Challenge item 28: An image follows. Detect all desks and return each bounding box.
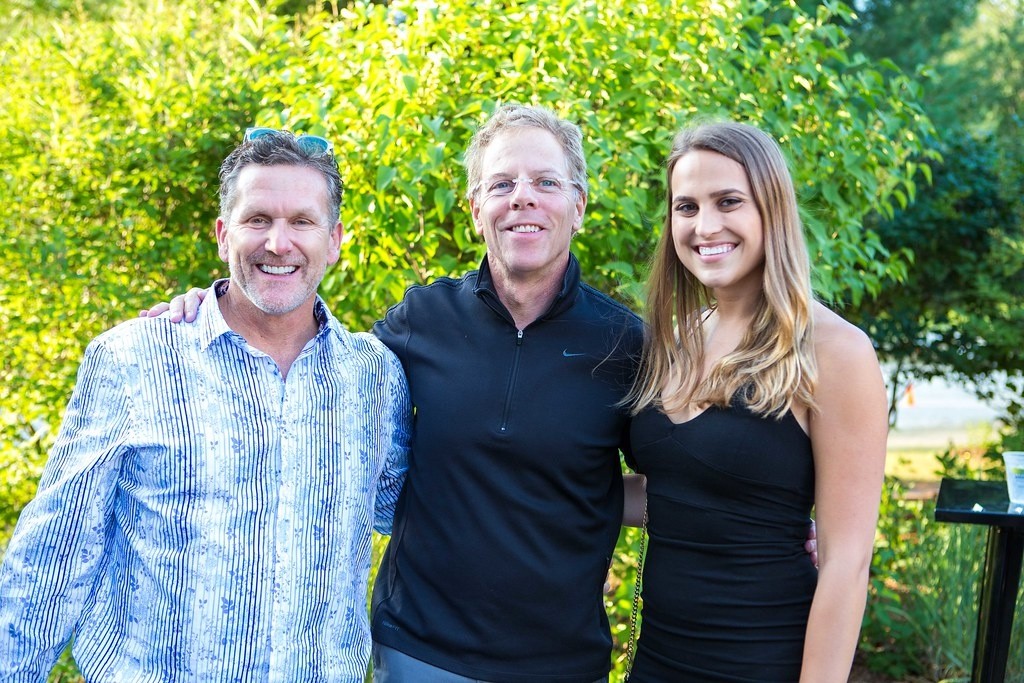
[934,476,1024,683]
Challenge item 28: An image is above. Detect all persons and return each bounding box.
[620,122,888,683]
[140,103,818,683]
[0,128,414,683]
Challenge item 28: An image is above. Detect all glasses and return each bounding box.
[474,173,581,195]
[243,127,334,163]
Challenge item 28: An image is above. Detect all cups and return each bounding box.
[1002,452,1024,503]
